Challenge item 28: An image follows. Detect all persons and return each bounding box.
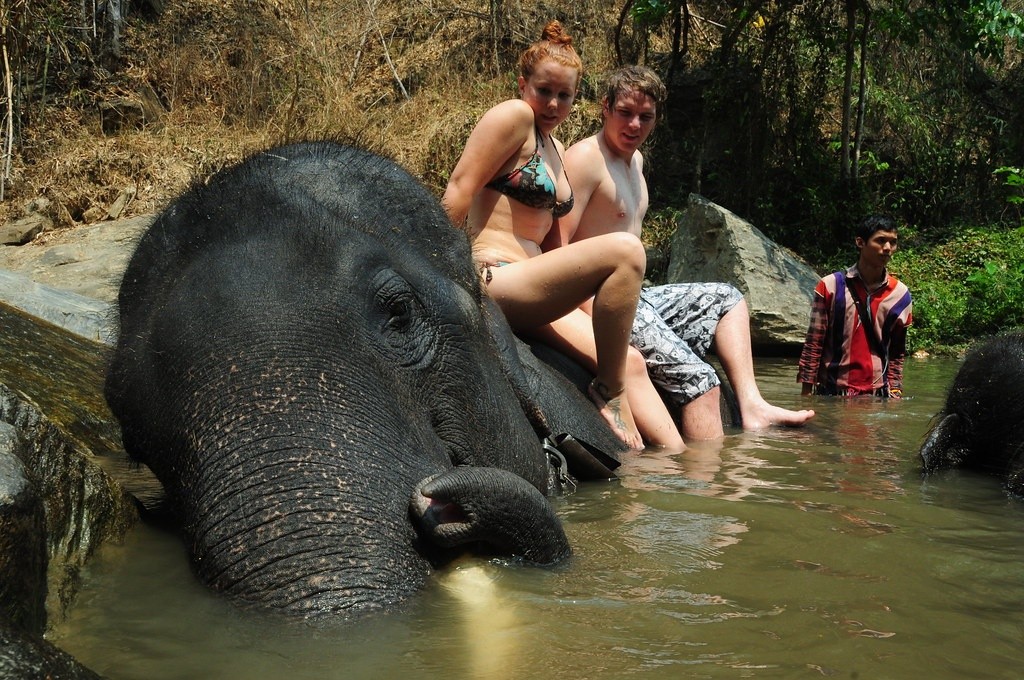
[438,21,689,455]
[538,63,814,442]
[795,210,915,402]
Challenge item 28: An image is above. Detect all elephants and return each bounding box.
[103,126,1023,640]
